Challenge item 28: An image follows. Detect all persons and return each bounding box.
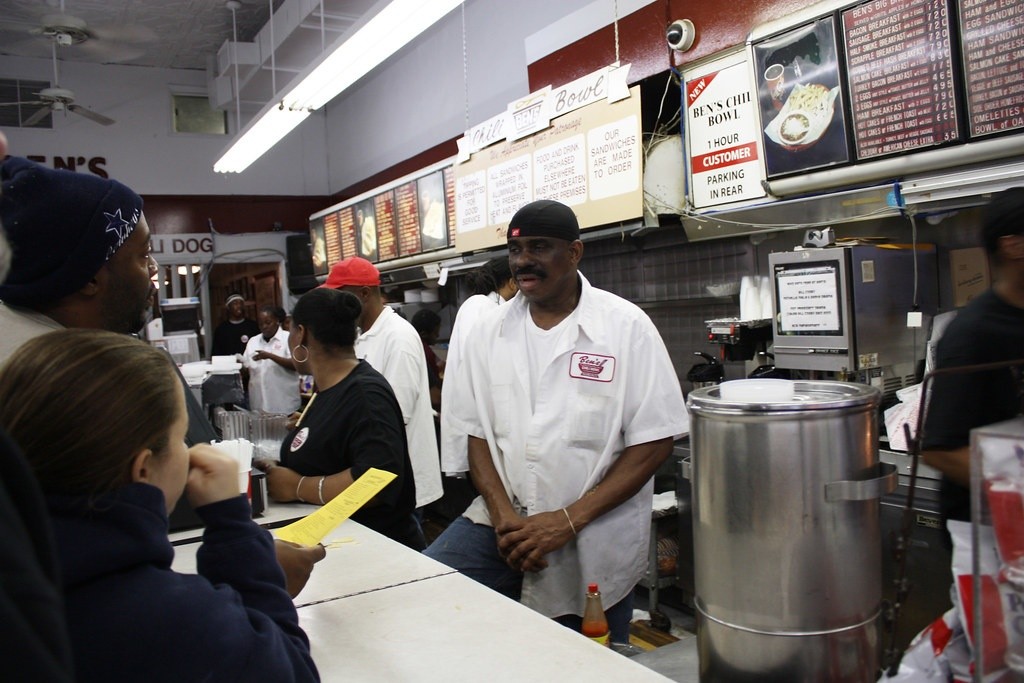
[205,255,519,554]
[0,157,325,601]
[918,185,1024,609]
[419,200,691,645]
[1,328,321,683]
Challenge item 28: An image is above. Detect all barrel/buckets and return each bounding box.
[684,378,901,634]
[693,596,888,683]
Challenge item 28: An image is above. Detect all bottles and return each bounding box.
[581,585,611,649]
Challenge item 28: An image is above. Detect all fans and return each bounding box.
[0,37,117,126]
[3,10,154,69]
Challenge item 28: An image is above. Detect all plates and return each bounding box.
[404,286,439,305]
[779,112,812,144]
[361,217,376,256]
[422,202,445,240]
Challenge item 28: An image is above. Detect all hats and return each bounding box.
[309,257,381,291]
[225,291,245,310]
[0,132,144,306]
[507,200,580,241]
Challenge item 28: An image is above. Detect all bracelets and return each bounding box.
[319,475,325,505]
[296,475,306,503]
[562,507,577,536]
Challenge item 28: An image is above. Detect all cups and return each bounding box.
[237,470,253,512]
[764,63,787,112]
[421,191,430,212]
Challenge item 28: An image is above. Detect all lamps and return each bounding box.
[209,0,466,174]
